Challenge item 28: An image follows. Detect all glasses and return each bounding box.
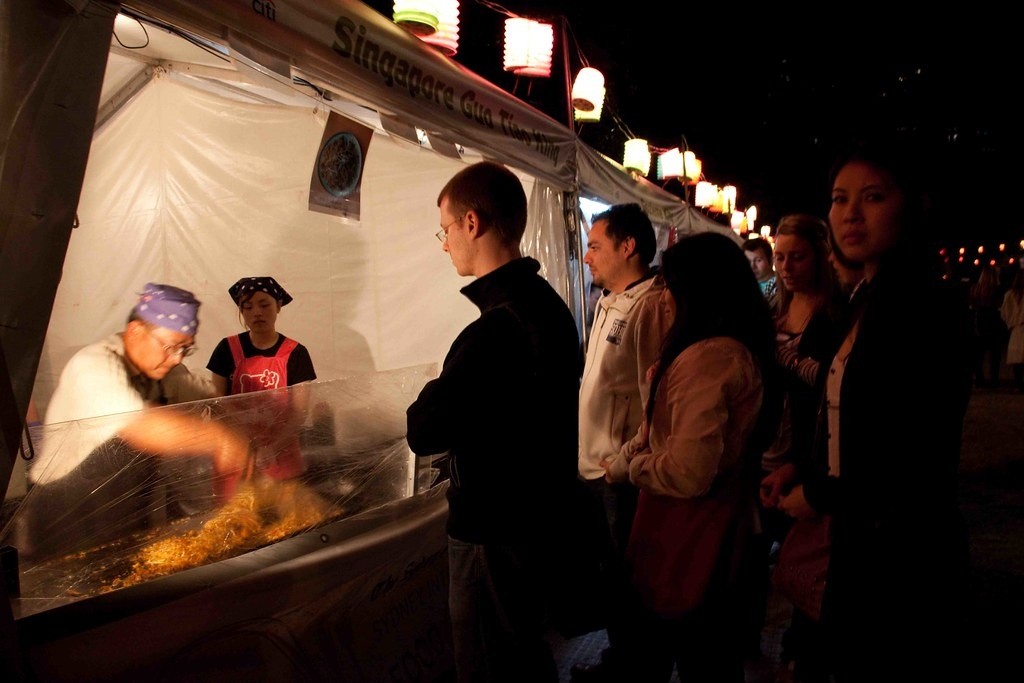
[143,326,197,357]
[435,214,469,244]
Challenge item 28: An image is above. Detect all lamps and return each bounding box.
[623,138,771,240]
[571,67,606,124]
[502,17,553,78]
[392,1,460,57]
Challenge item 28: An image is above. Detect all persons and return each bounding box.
[28,281,252,566]
[802,146,977,683]
[408,163,1024,683]
[207,278,316,510]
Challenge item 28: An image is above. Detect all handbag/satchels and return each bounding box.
[620,481,741,616]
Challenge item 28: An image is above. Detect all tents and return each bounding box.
[570,138,752,359]
[0,0,577,683]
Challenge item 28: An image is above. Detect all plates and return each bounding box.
[319,132,361,197]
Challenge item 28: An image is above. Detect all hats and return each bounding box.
[137,282,200,334]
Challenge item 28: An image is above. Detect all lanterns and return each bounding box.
[624,138,652,177]
[393,0,460,55]
[504,18,554,77]
[572,67,606,122]
[657,147,757,235]
